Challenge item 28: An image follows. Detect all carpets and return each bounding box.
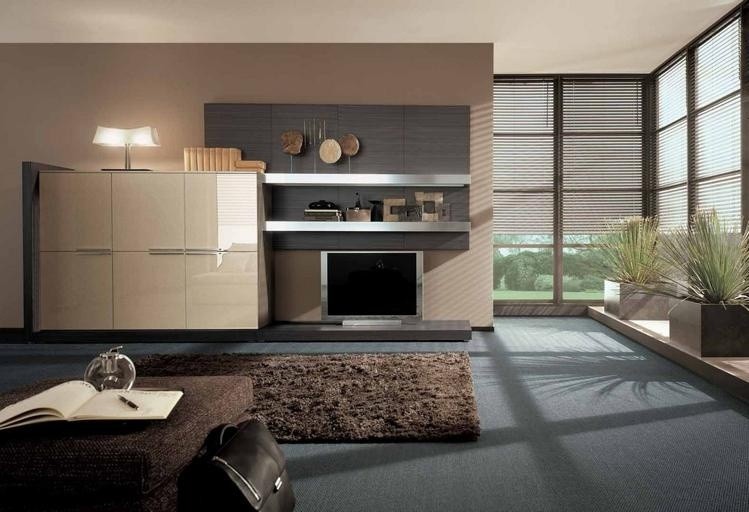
[121,351,481,444]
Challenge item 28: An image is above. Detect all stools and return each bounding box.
[0,374,254,512]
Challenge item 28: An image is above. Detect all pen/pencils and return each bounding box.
[117,394,139,410]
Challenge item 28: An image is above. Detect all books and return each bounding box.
[235,166,265,173]
[0,379,184,431]
[183,147,242,172]
[234,159,266,169]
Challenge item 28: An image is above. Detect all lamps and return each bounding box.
[92,125,162,171]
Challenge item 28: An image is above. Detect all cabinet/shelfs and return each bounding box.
[262,173,473,233]
[37,170,272,332]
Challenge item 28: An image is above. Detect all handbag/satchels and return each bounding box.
[208,417,296,512]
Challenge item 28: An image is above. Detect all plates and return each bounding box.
[318,134,359,164]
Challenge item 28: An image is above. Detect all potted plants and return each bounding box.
[585,207,748,358]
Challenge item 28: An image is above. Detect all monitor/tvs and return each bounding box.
[320,250,423,326]
